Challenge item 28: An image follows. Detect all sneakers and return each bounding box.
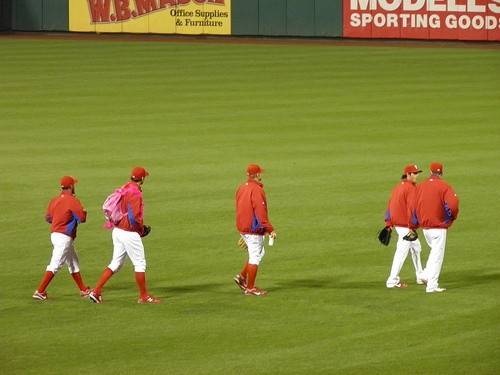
[234,274,247,291]
[394,283,406,288]
[89,291,103,304]
[426,286,446,292]
[245,286,267,295]
[138,296,160,303]
[420,276,427,284]
[79,289,93,299]
[32,290,46,300]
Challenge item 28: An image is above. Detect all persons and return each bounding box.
[384,165,424,289]
[88,167,160,303]
[32,176,94,300]
[409,162,459,292]
[233,164,277,295]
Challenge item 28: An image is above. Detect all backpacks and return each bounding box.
[103,187,140,227]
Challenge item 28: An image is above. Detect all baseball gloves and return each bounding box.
[142,225,152,237]
[237,237,248,250]
[402,230,419,242]
[377,226,392,246]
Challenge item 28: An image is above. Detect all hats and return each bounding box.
[60,176,78,188]
[405,166,422,175]
[429,162,443,173]
[131,167,149,180]
[247,165,264,175]
